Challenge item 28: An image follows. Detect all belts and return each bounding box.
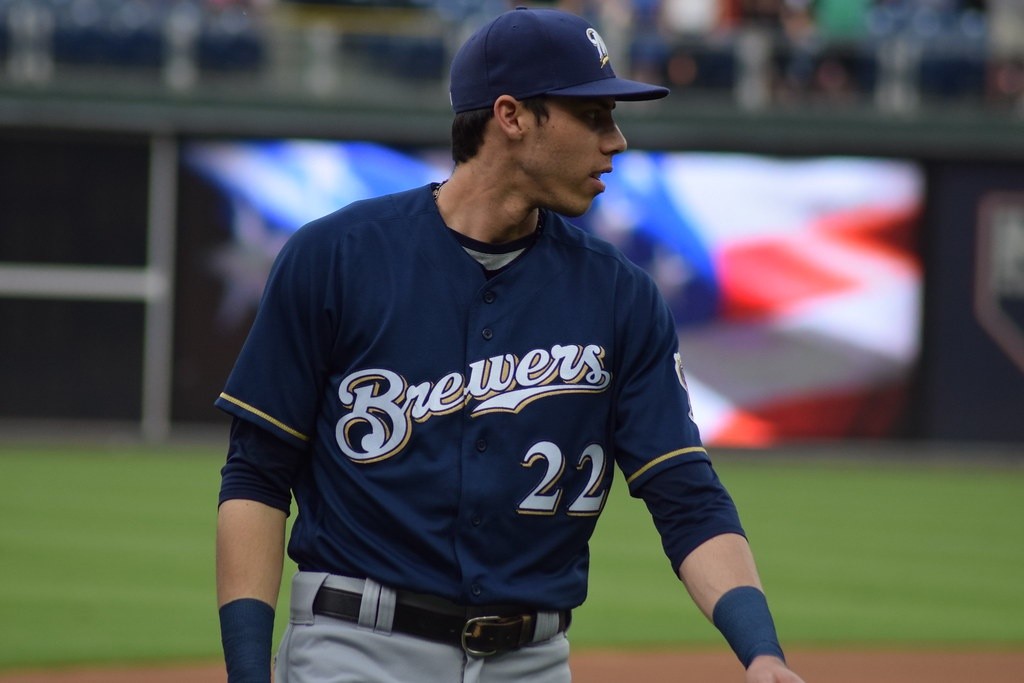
[317,587,573,657]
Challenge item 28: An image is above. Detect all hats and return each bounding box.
[448,7,671,108]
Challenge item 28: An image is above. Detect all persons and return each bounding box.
[214,9,804,683]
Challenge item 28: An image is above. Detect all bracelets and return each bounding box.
[710,585,786,670]
[219,598,275,683]
